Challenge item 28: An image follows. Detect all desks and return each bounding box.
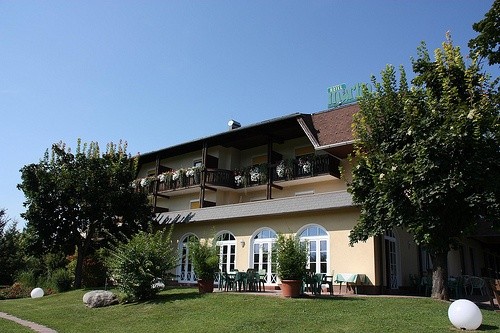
[227,273,258,291]
[334,273,369,296]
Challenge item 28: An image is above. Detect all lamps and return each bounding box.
[240,240,245,248]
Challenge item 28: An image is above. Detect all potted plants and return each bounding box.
[55,268,73,292]
[183,226,223,294]
[273,227,311,297]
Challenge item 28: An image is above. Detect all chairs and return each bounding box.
[409,271,487,299]
[305,271,333,295]
[218,269,267,292]
